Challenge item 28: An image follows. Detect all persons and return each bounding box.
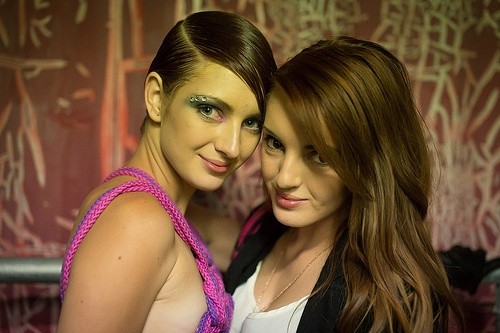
[56,10,276,333]
[220,35,464,333]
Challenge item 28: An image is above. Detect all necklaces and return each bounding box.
[252,241,338,312]
